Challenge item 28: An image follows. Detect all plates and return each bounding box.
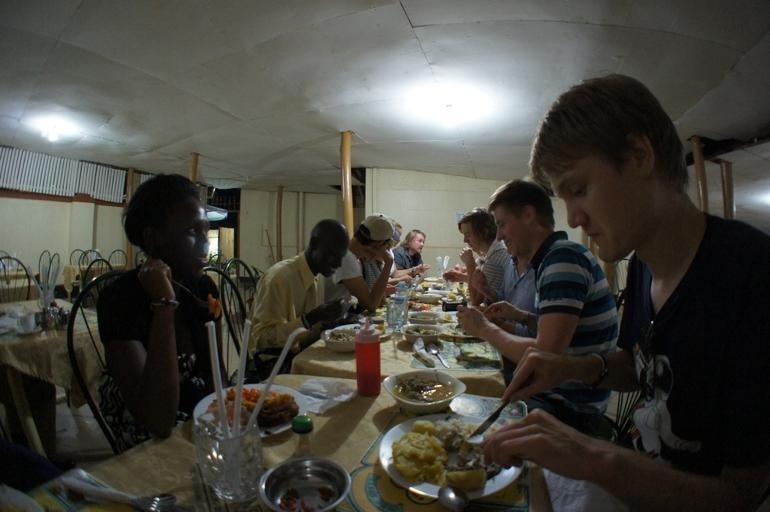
[17,327,43,335]
[379,416,529,503]
[192,385,308,440]
[323,278,490,368]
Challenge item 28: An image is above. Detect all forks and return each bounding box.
[60,475,176,512]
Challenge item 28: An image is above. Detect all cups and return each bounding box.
[19,313,36,332]
[192,405,266,504]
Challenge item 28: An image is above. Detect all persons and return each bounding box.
[96,173,229,447]
[319,214,396,322]
[454,249,485,273]
[362,218,431,296]
[472,244,537,339]
[455,177,618,434]
[250,218,354,382]
[477,73,770,512]
[442,207,511,307]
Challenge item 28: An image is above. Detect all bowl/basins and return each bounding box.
[258,455,352,512]
[383,369,468,415]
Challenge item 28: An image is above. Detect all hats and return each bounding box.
[361,213,394,241]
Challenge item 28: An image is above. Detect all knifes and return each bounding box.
[468,390,519,437]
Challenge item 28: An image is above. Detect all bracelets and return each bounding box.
[583,348,611,393]
[518,310,533,327]
[298,310,311,329]
[148,295,182,314]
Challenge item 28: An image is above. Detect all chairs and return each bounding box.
[201,270,248,385]
[0,246,147,303]
[219,258,257,360]
[588,288,644,450]
[66,269,136,456]
